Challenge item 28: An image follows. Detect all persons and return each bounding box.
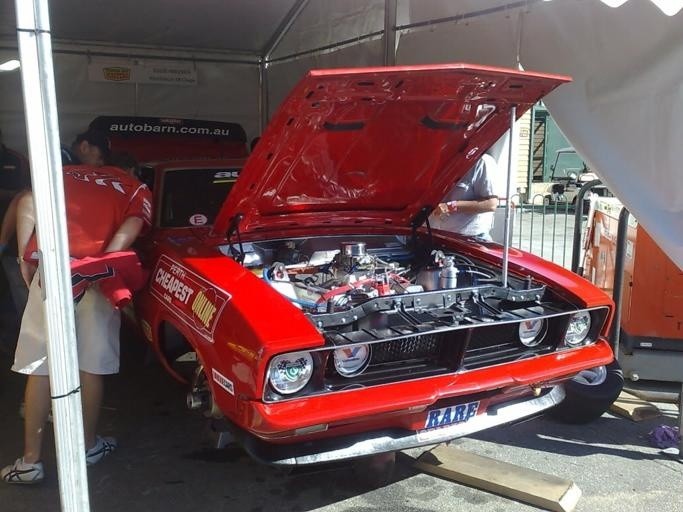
[0,154,154,484]
[0,130,112,423]
[431,153,502,244]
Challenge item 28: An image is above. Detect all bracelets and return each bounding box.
[447,199,459,217]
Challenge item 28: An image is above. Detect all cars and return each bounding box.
[71,62,624,478]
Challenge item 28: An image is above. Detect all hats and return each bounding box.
[71,129,111,148]
[61,148,79,164]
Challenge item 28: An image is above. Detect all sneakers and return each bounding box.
[85,436,117,466]
[20,401,52,423]
[1,457,45,485]
